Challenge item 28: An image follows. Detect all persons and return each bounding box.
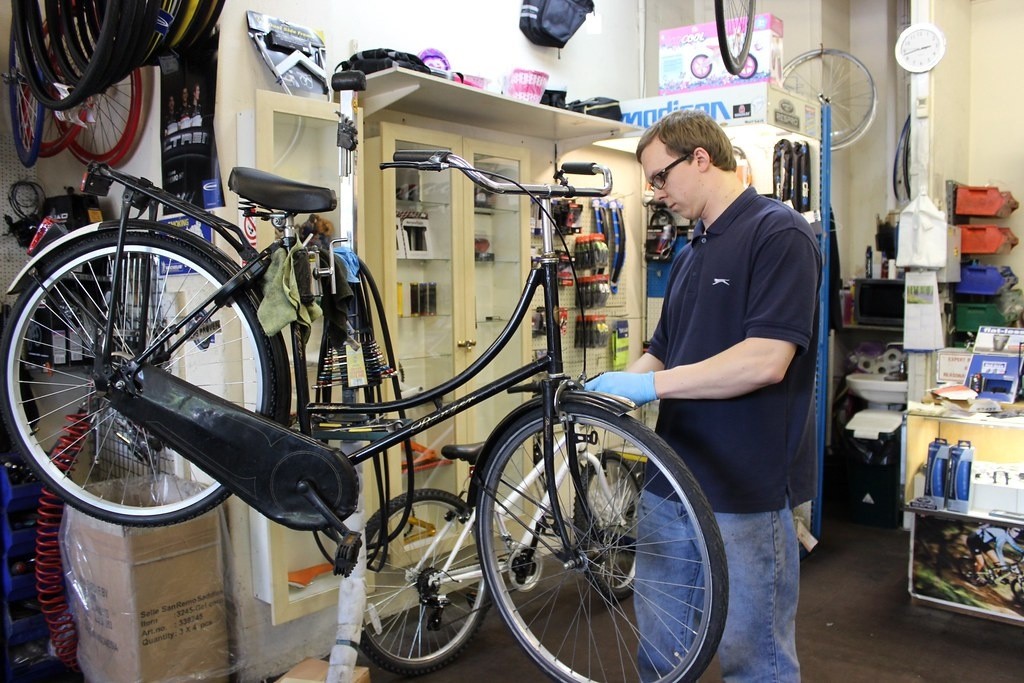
[967,525,1024,581]
[581,108,824,683]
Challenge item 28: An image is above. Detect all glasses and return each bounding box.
[649,145,713,190]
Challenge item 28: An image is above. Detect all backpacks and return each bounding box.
[519,0,595,60]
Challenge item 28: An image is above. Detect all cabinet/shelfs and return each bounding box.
[254,67,643,626]
[901,347,1024,525]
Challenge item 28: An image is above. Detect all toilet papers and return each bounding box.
[856,348,901,377]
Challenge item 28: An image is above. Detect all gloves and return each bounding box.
[582,370,659,410]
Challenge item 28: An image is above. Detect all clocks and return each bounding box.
[894,24,946,73]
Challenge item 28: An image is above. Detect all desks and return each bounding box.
[825,324,903,454]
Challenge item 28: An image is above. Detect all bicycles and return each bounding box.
[0,149,728,683]
[688,27,758,80]
[956,553,1024,607]
[352,379,642,678]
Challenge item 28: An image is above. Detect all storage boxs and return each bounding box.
[955,302,1011,332]
[658,14,784,95]
[273,658,371,683]
[955,187,1018,218]
[65,473,230,683]
[955,259,1017,295]
[960,224,1021,255]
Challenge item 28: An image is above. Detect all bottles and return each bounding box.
[925,438,951,509]
[946,440,973,512]
